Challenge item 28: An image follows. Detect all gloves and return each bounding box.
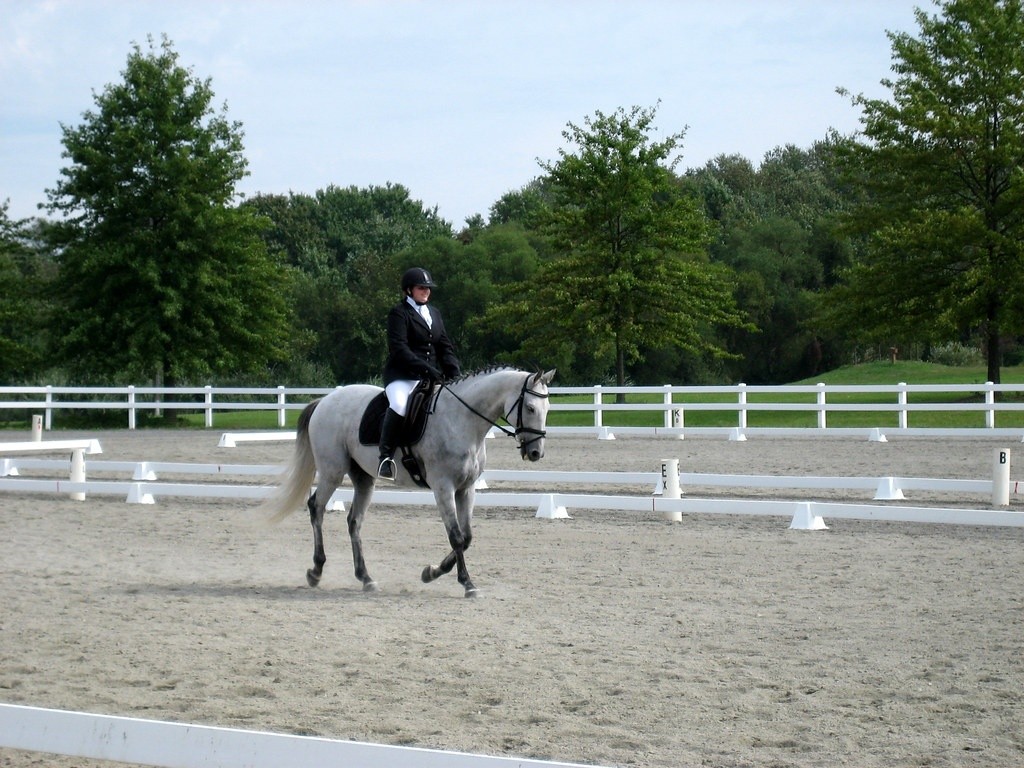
[429,367,443,380]
[443,355,460,375]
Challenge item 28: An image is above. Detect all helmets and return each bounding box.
[401,267,437,306]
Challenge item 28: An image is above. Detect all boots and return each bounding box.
[377,407,406,478]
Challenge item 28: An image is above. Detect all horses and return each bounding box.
[248,366,557,598]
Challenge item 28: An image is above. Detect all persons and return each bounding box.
[377,267,461,478]
